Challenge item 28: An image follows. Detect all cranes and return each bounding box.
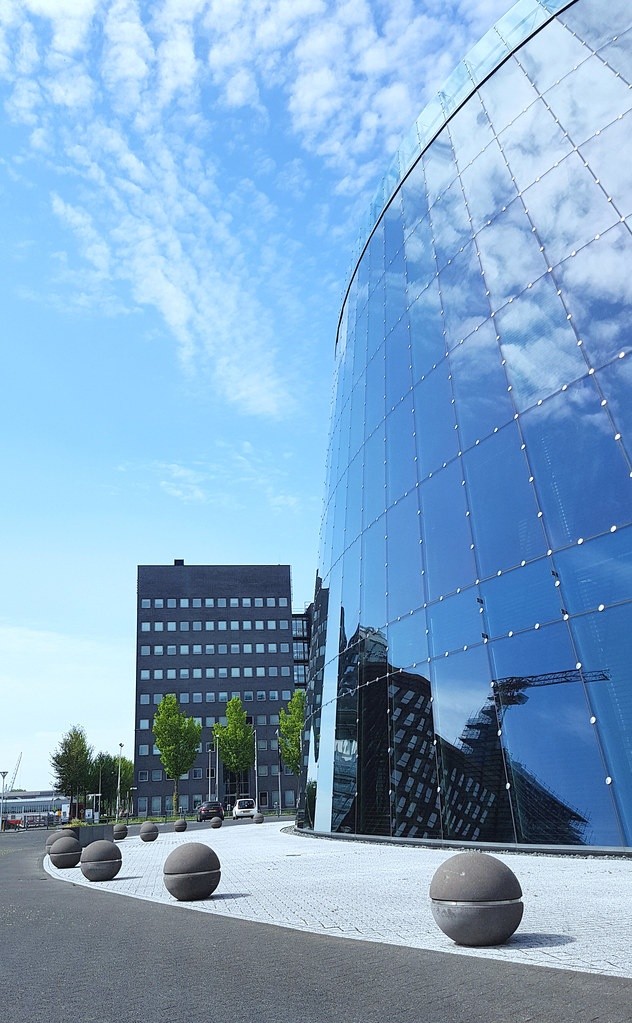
[487,665,611,736]
[7,752,24,792]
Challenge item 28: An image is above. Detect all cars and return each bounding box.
[196,800,225,822]
[231,799,255,820]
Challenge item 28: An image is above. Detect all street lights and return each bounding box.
[118,742,124,806]
[215,734,219,801]
[208,749,212,802]
[0,771,9,820]
